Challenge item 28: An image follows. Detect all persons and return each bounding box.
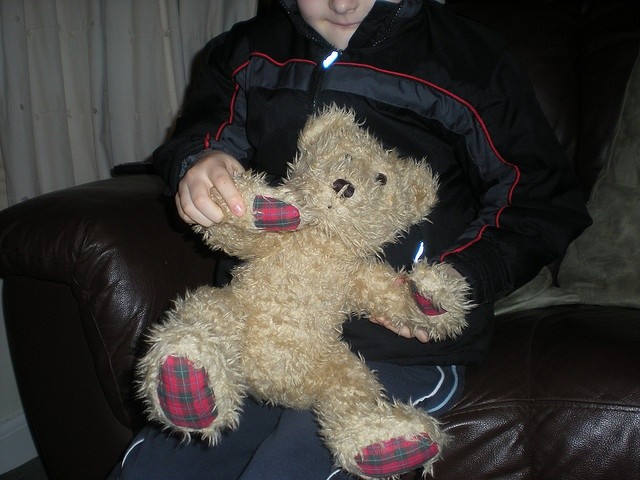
[111,0,593,480]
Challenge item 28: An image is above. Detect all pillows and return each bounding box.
[555,49,640,304]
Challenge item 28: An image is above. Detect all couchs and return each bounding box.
[1,2,640,480]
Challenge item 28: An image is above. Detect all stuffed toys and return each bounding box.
[137,104,478,480]
